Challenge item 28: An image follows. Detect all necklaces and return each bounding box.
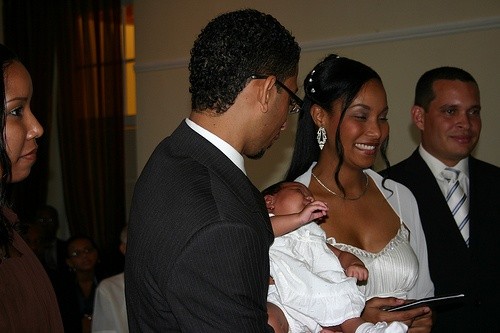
[311,171,369,201]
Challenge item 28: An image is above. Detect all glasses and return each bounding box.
[68,246,93,258]
[252,74,304,114]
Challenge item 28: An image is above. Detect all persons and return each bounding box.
[122,8,309,333]
[0,42,65,333]
[19,205,103,333]
[88,226,129,333]
[377,66,500,333]
[285,52,436,333]
[260,179,409,333]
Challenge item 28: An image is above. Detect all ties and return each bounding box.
[440,168,471,249]
